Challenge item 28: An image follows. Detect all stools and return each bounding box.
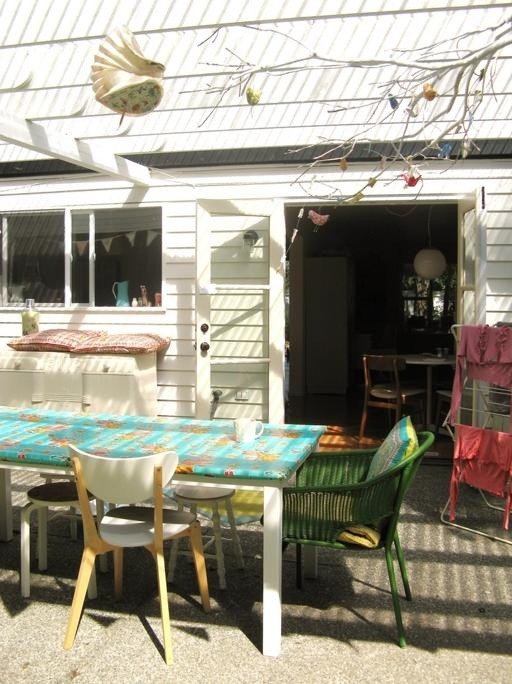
[40,472,136,540]
[20,482,108,600]
[166,485,245,590]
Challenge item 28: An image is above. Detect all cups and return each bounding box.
[234,416,263,442]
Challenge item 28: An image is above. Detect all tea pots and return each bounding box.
[112,279,131,308]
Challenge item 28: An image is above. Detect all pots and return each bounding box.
[414,346,450,358]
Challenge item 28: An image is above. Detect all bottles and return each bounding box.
[132,283,160,306]
[21,297,39,334]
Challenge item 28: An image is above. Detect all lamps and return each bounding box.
[415,205,446,279]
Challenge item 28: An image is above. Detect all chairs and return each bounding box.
[63,444,210,666]
[359,354,452,441]
[260,430,434,649]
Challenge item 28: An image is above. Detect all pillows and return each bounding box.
[8,329,171,354]
[366,415,419,481]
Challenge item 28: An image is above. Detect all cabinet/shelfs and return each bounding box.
[305,257,355,395]
[0,351,158,417]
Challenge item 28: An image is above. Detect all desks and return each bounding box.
[0,406,328,656]
